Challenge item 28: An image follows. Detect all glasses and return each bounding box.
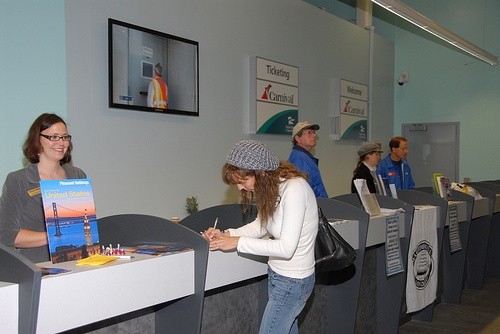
[40,133,72,141]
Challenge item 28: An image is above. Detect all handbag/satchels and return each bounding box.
[313,208,356,277]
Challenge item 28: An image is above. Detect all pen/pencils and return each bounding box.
[214,217,218,229]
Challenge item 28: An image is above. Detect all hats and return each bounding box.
[357,142,384,156]
[292,122,320,141]
[226,140,280,171]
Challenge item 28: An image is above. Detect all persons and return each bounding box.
[284,121,330,198]
[147,63,168,109]
[200,140,318,334]
[352,143,384,194]
[376,137,415,191]
[0,112,86,264]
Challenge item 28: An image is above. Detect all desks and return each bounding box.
[0,193,500,334]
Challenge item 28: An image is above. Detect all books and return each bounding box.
[432,173,451,201]
